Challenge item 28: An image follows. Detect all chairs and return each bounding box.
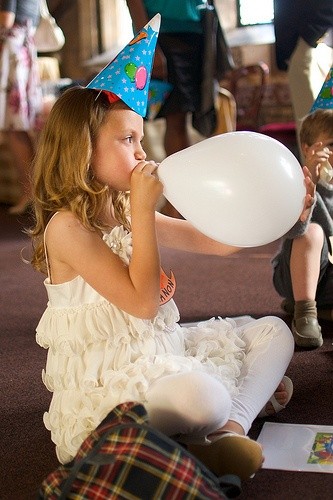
[227,62,270,133]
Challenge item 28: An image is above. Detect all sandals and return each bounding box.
[192,430,263,479]
[258,376,293,417]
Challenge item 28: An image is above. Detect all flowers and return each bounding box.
[102,225,133,265]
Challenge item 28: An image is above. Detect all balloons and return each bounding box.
[155,130,307,248]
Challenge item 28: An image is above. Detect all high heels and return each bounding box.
[8,198,28,213]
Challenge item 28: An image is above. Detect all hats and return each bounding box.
[85,12,162,118]
[308,65,333,112]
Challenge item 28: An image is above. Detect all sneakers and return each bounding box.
[292,316,324,347]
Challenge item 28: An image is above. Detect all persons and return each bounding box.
[273,0,333,166]
[0,0,46,215]
[126,0,236,220]
[20,13,295,481]
[272,109,333,348]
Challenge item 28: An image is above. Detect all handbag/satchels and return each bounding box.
[32,0,65,52]
